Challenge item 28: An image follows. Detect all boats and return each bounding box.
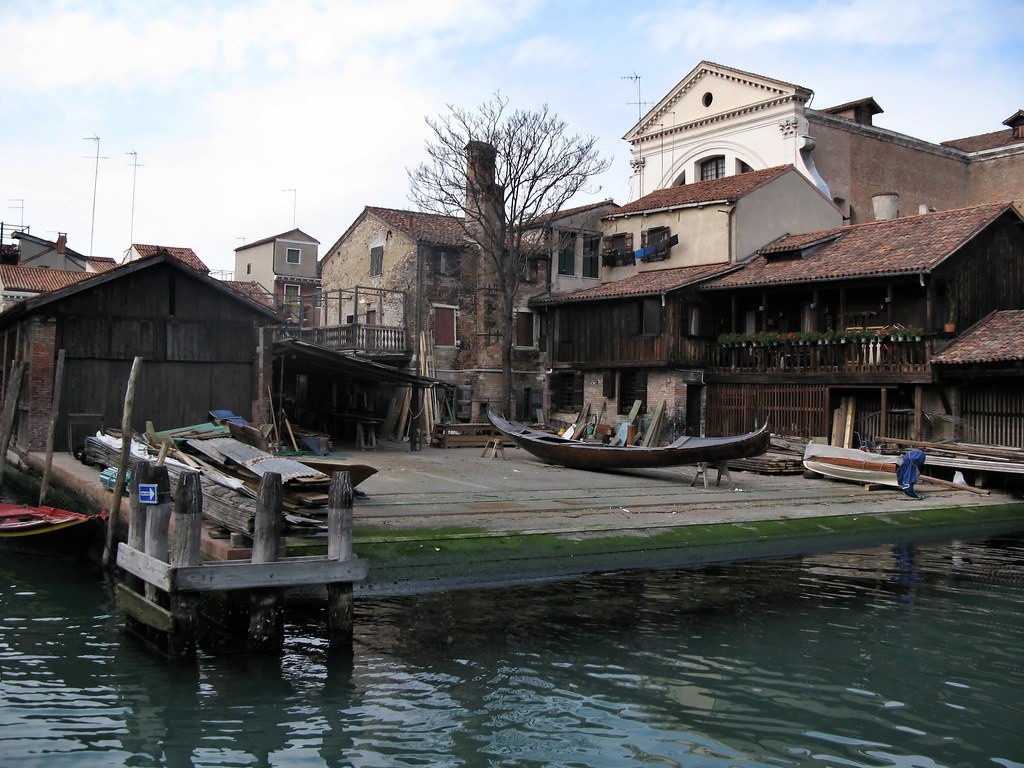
[484,397,772,471]
[802,442,921,488]
[0,504,109,550]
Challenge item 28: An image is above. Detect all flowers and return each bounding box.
[911,325,924,336]
[898,326,907,335]
[749,333,758,342]
[876,328,887,337]
[769,332,780,341]
[758,332,768,341]
[903,325,915,335]
[889,326,897,336]
[787,333,798,339]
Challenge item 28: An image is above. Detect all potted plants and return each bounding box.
[816,329,870,345]
[804,333,813,345]
[799,332,804,345]
[945,299,957,332]
[717,333,749,347]
[870,331,876,342]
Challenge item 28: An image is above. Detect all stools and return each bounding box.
[481,438,505,461]
[690,459,734,490]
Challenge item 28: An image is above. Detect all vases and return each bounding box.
[884,296,890,302]
[878,337,882,341]
[753,342,756,346]
[898,335,904,341]
[809,302,817,309]
[890,335,897,341]
[773,341,777,345]
[759,305,765,311]
[761,341,764,346]
[792,340,796,345]
[916,336,920,341]
[907,335,912,341]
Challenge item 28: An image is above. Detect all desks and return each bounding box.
[309,408,385,448]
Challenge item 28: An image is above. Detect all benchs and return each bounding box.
[527,433,557,440]
[560,442,605,446]
